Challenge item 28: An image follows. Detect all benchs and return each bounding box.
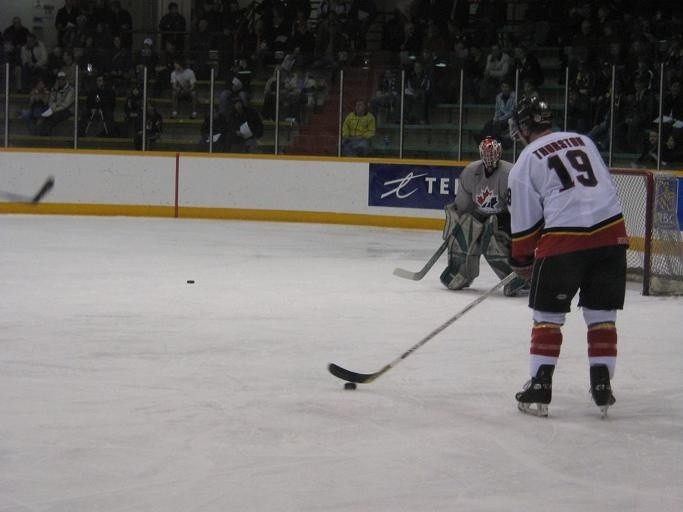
[0,0,682,170]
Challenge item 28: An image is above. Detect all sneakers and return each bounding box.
[515,362,554,404]
[589,363,617,407]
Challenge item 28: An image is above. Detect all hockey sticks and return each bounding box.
[0,175,54,203]
[393,205,476,281]
[327,270,517,382]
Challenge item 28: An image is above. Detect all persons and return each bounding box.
[374,1,682,167]
[505,94,631,407]
[2,1,375,157]
[439,136,530,296]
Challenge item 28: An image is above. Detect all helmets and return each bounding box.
[479,136,502,162]
[510,92,552,135]
